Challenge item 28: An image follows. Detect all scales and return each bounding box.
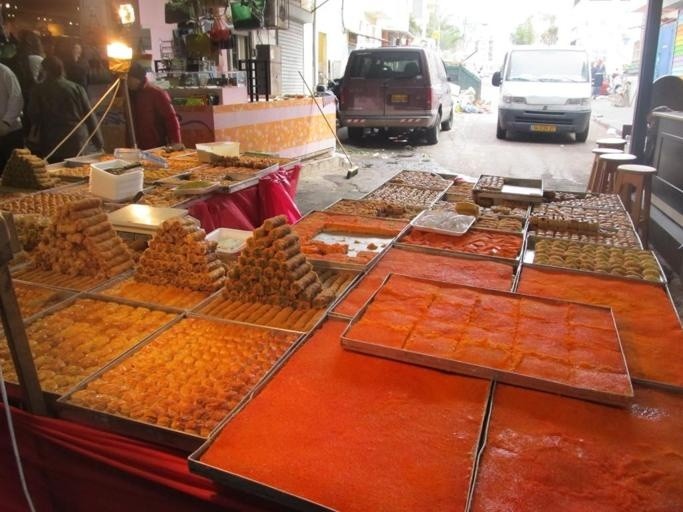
[106,203,189,244]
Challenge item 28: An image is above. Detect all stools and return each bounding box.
[585,138,658,249]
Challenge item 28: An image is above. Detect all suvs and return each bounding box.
[339,47,453,144]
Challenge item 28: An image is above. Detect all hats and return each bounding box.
[128,64,145,78]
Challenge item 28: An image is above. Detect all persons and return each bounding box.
[122,60,181,150]
[0,22,112,176]
[591,55,631,107]
[457,86,486,113]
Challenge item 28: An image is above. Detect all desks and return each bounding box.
[166,85,248,106]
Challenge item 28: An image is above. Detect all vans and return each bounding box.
[492,44,593,142]
